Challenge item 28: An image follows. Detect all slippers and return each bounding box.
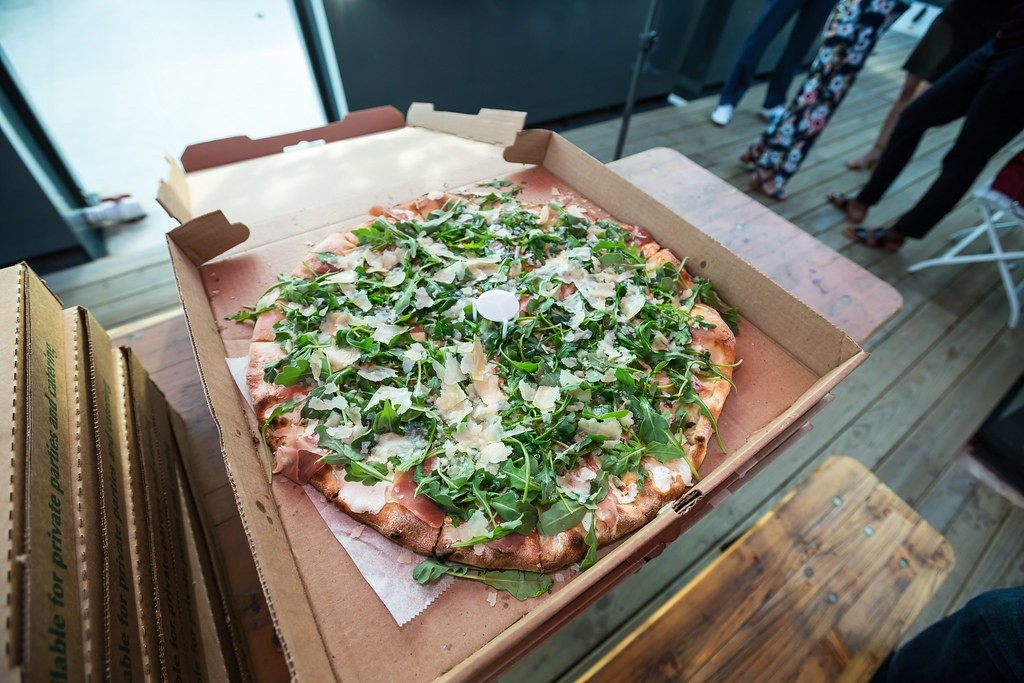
[828,192,861,225]
[841,227,903,253]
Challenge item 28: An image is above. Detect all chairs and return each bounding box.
[903,134,1023,329]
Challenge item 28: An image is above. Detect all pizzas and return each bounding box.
[221,195,743,572]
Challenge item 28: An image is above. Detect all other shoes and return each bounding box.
[761,105,785,119]
[711,104,733,125]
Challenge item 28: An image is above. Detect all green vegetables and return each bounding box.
[411,557,554,601]
[475,178,525,195]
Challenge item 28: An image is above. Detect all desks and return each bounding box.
[106,147,903,682]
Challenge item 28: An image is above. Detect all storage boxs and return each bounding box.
[154,102,902,683]
[0,262,255,683]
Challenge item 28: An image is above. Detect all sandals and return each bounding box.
[846,142,885,172]
[740,148,760,164]
[751,169,787,201]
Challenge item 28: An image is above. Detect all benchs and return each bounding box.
[575,456,954,682]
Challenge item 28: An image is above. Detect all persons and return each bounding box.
[876,585,1024,683]
[711,0,914,200]
[826,0,1024,252]
